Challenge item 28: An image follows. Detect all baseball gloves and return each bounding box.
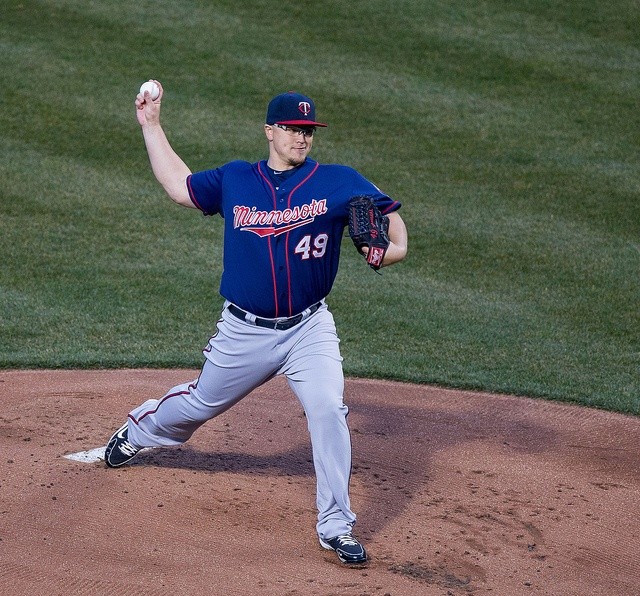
[344,194,390,276]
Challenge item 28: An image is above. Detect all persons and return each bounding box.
[104,78,408,563]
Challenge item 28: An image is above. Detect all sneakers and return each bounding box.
[105,398,158,469]
[319,530,367,563]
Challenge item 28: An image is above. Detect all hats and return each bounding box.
[266,90,328,127]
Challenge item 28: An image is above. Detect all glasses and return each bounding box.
[274,123,312,137]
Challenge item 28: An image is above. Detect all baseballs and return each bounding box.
[139,82,160,100]
